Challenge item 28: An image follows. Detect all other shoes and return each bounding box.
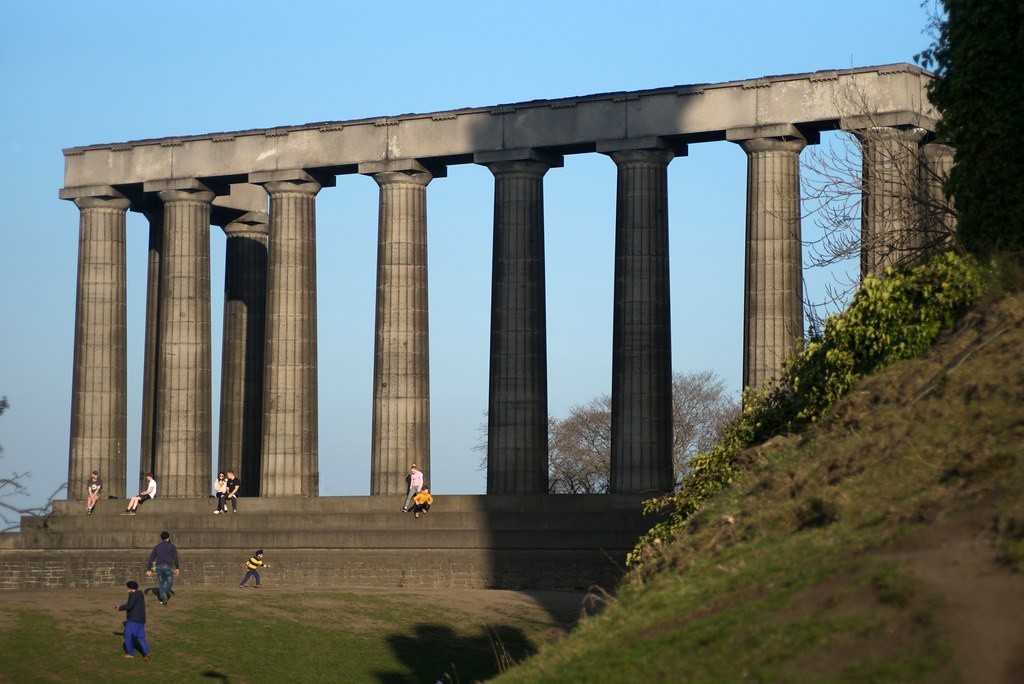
[220,509,226,515]
[421,508,427,514]
[88,507,92,515]
[400,508,407,512]
[239,584,244,588]
[162,593,167,605]
[160,601,163,604]
[213,511,219,514]
[144,654,151,661]
[224,503,228,511]
[414,512,418,519]
[120,511,135,516]
[233,509,237,513]
[256,584,263,588]
[121,653,134,659]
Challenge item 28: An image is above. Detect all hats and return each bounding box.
[127,581,139,591]
[160,531,170,540]
[255,550,264,555]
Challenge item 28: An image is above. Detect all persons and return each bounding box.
[121,472,157,516]
[213,470,240,514]
[239,549,272,588]
[146,530,179,607]
[401,466,423,512]
[87,470,103,514]
[114,580,151,663]
[413,486,434,518]
